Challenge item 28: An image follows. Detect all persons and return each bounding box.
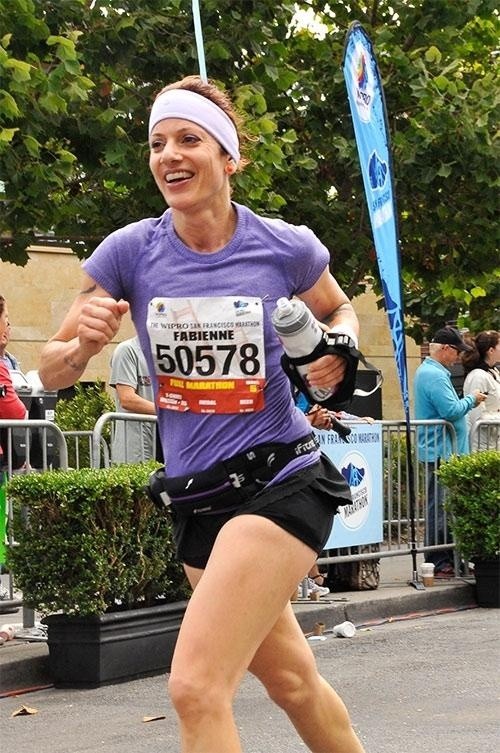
[1,317,19,370]
[321,408,375,427]
[292,387,333,602]
[413,324,490,582]
[38,71,363,751]
[109,334,158,463]
[1,295,28,421]
[464,328,499,458]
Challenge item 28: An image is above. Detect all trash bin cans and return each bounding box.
[1,369,57,469]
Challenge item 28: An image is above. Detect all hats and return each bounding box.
[430,327,472,351]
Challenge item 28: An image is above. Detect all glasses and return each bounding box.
[448,344,462,355]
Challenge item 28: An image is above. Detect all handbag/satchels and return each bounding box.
[323,544,381,588]
[147,443,288,514]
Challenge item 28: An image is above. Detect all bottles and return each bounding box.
[271,297,337,402]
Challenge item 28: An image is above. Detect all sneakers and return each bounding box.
[297,576,338,599]
[434,562,474,577]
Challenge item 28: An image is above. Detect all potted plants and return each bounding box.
[0,458,193,689]
[434,449,499,608]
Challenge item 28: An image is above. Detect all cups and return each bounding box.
[419,562,435,587]
[333,621,356,638]
